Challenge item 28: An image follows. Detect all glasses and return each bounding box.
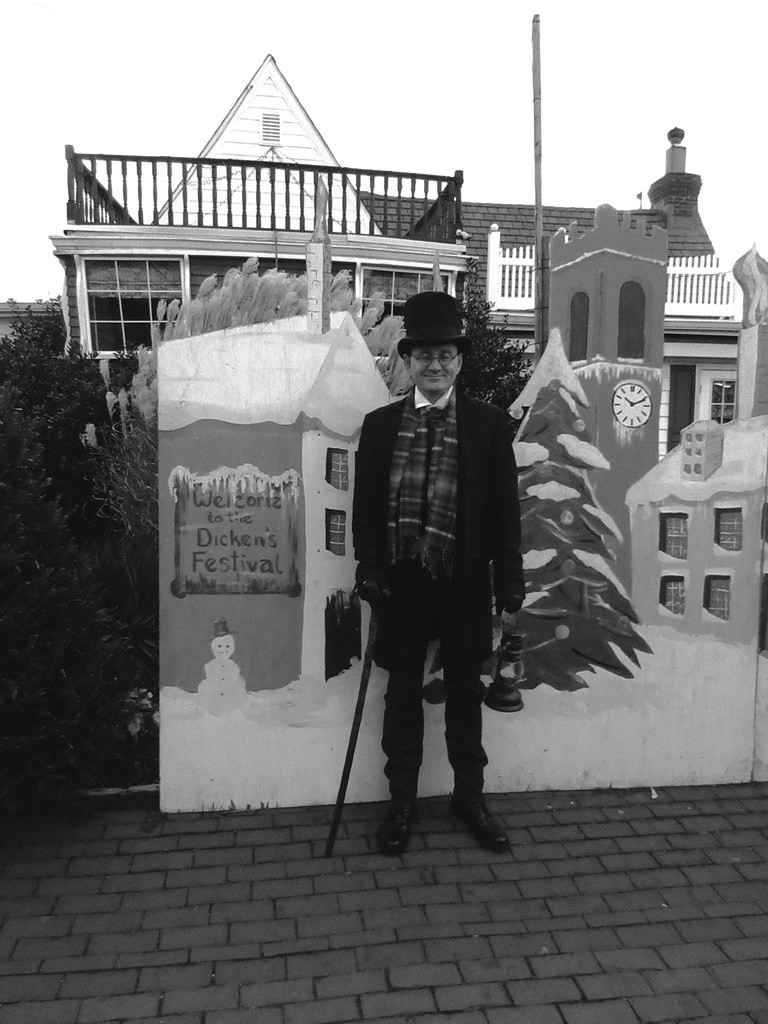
[409,353,460,367]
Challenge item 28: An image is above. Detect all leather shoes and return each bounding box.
[377,796,417,855]
[449,797,510,850]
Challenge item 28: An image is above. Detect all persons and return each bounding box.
[351,291,527,853]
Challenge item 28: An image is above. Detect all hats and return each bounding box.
[397,292,471,359]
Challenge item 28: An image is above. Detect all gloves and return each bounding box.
[496,592,525,615]
[356,579,400,613]
